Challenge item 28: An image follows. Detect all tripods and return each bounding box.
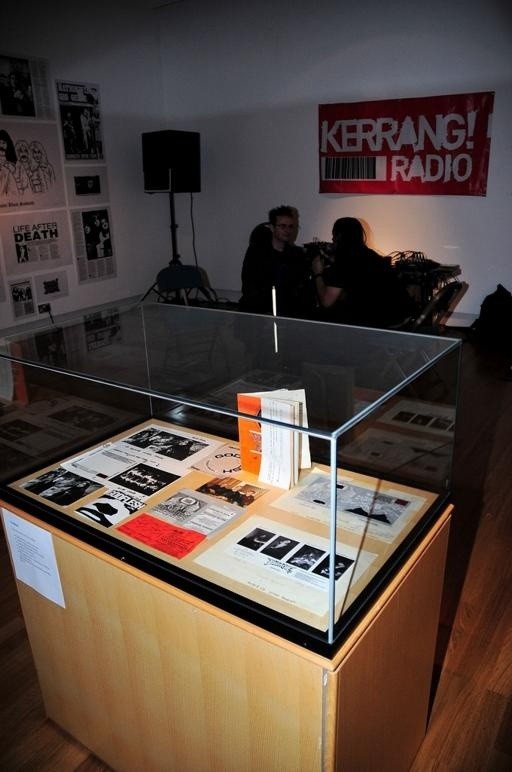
[141,196,215,309]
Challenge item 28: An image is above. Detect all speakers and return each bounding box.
[142,129,201,194]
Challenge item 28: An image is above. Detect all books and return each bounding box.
[237,387,311,491]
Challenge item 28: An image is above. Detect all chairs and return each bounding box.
[369,281,469,399]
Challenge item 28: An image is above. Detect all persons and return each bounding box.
[195,482,256,508]
[288,553,318,567]
[321,562,346,575]
[261,539,300,559]
[163,438,195,462]
[120,467,166,492]
[84,215,110,258]
[312,217,397,326]
[231,205,312,355]
[239,531,267,549]
[24,467,90,506]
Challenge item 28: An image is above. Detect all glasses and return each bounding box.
[274,223,293,229]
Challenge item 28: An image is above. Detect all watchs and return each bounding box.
[309,274,322,280]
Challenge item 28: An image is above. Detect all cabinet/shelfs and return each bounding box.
[0,300,464,771]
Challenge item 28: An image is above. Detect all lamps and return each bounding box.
[140,130,218,304]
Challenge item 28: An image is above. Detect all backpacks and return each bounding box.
[466,283,512,352]
